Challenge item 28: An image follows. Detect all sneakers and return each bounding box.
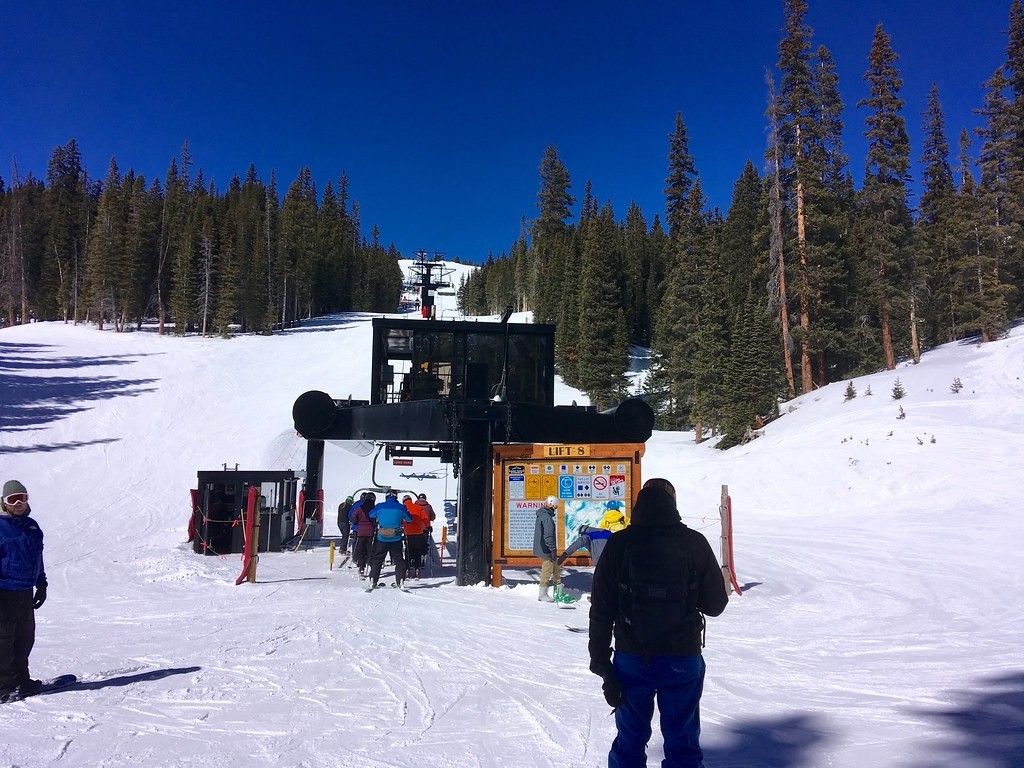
[340,550,425,589]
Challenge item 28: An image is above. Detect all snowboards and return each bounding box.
[564,624,590,633]
[0,674,77,704]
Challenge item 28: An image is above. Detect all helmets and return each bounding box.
[419,493,426,500]
[607,500,619,511]
[545,496,558,508]
[365,492,376,502]
[346,496,354,505]
[386,489,397,500]
[402,495,411,503]
[578,525,588,537]
[361,493,368,499]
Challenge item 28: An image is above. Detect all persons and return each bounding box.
[0,480,47,703]
[533,496,562,603]
[401,296,407,312]
[558,523,613,566]
[369,489,412,588]
[415,297,420,311]
[586,477,729,768]
[598,500,632,533]
[349,492,376,578]
[402,493,435,580]
[337,496,353,554]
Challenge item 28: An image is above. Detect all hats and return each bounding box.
[643,477,676,501]
[3,480,27,499]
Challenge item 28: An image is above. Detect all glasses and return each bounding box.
[3,493,29,505]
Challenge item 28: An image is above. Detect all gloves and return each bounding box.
[551,551,557,561]
[32,584,47,609]
[589,656,613,680]
[557,553,568,565]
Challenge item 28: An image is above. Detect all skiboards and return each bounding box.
[405,577,419,582]
[365,583,410,593]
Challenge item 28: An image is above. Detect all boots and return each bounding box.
[2,690,22,703]
[538,585,555,602]
[553,583,563,597]
[20,671,43,695]
